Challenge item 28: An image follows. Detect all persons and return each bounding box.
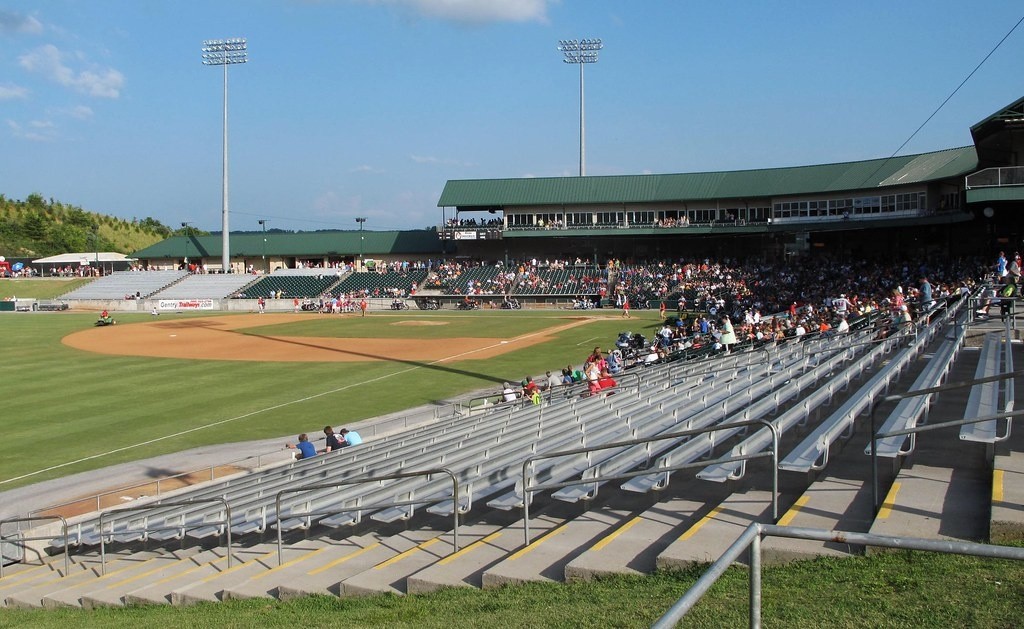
[285,426,362,459]
[10,265,34,278]
[101,309,109,319]
[49,264,103,277]
[373,280,418,298]
[378,259,432,273]
[298,260,355,272]
[294,286,369,317]
[427,257,652,319]
[501,346,622,406]
[446,209,859,230]
[644,252,1024,365]
[236,292,246,298]
[257,297,264,314]
[125,292,141,300]
[130,263,156,271]
[270,288,288,299]
[248,265,262,276]
[153,307,158,313]
[189,261,208,274]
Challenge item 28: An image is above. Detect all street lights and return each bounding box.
[259,220,265,275]
[356,218,365,273]
[202,38,247,273]
[558,38,603,178]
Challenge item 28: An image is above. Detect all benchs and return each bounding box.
[46,286,1015,551]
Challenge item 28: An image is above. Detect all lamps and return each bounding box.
[984,206,993,217]
